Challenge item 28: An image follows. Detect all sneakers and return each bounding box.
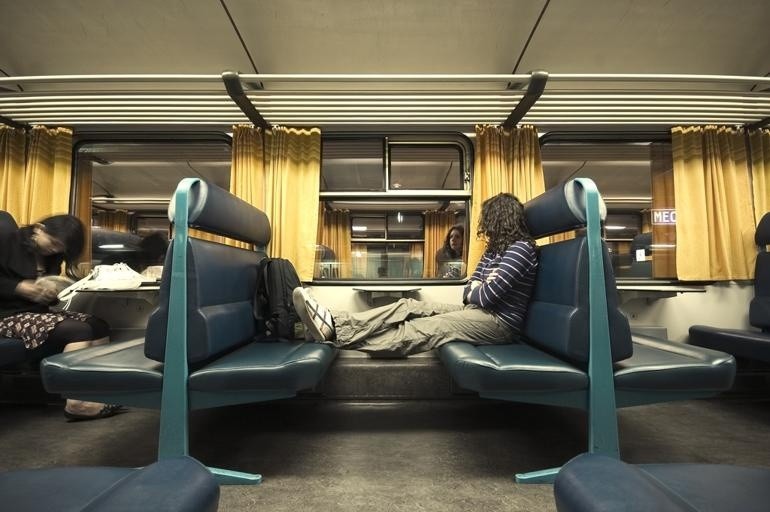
[292,286,336,343]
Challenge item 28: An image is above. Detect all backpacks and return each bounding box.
[252,257,308,344]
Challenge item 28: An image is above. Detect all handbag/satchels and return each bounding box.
[56,262,143,301]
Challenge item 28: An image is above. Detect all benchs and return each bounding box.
[689,323,769,363]
[1,457,221,511]
[41,176,339,487]
[555,453,769,511]
[436,177,736,483]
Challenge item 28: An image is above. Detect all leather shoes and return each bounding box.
[62,404,123,420]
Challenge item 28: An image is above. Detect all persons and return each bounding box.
[436,222,464,265]
[1,215,121,418]
[293,193,539,352]
